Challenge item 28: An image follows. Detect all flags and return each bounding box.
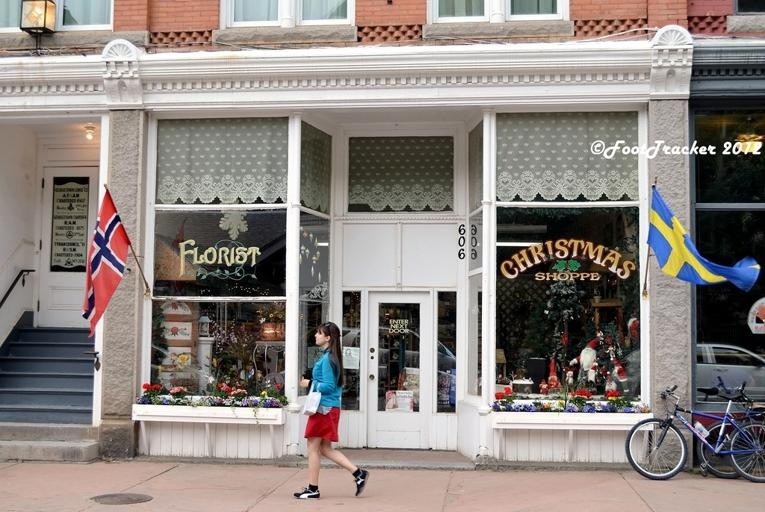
[80,190,132,340]
[643,184,763,295]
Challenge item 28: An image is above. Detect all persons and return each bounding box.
[292,322,369,499]
[567,337,599,389]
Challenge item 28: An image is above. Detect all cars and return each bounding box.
[603,343,765,398]
[283,326,456,397]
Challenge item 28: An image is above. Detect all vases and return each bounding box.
[492,412,653,430]
[132,404,285,425]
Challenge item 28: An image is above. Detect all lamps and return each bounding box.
[735,116,764,153]
[20,0,56,55]
[84,127,95,140]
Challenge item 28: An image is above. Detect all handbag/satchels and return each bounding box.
[302,392,321,416]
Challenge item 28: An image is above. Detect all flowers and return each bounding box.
[136,383,287,407]
[489,387,648,413]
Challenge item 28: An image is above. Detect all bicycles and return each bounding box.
[625,384,765,484]
[696,376,752,402]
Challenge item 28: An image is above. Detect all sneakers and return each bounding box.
[294,488,319,498]
[355,469,369,496]
